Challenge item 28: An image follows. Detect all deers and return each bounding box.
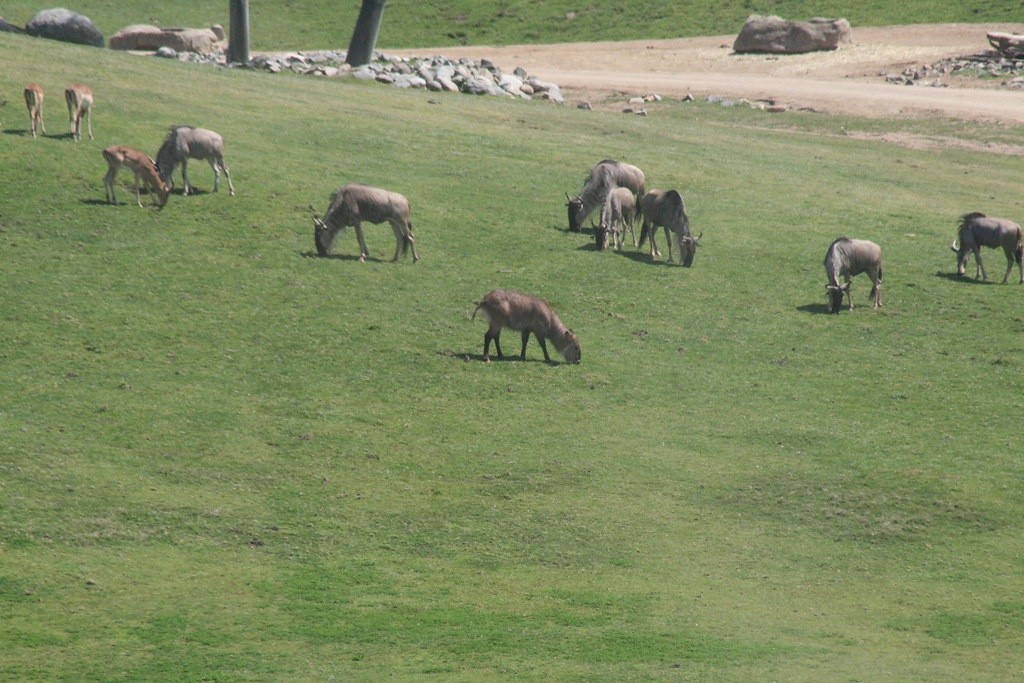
[64,81,95,143]
[102,145,174,210]
[24,82,47,140]
